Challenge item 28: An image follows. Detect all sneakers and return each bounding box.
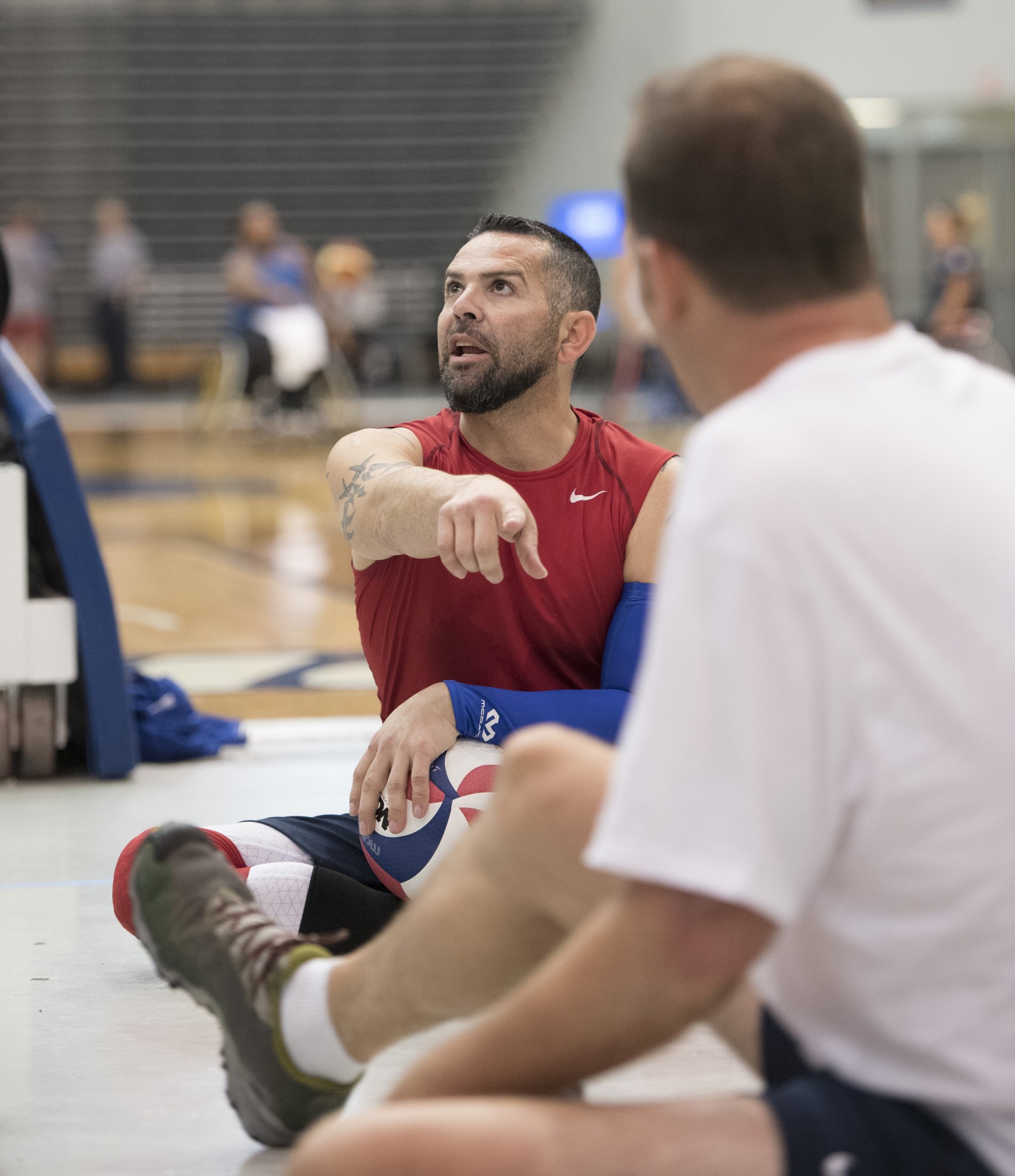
[128,823,366,1148]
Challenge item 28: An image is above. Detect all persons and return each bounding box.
[112,53,1015,1176]
[911,198,989,342]
[218,200,387,409]
[0,191,160,413]
